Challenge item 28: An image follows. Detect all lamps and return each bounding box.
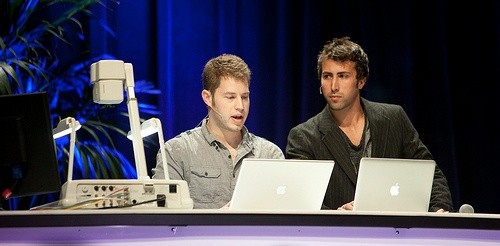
[51,117,82,208]
[127,117,169,179]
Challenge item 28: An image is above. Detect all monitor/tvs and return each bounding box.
[0,92,61,200]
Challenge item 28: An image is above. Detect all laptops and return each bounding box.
[229,158,334,209]
[351,158,437,212]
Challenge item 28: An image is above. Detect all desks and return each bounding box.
[1,208,500,246]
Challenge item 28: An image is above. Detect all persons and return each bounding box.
[151,54,286,210]
[284,37,455,215]
